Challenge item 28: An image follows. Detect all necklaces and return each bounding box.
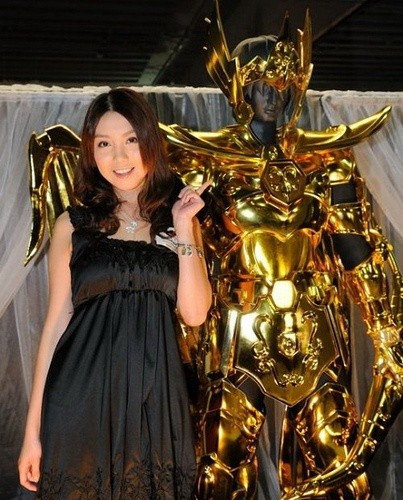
[113,203,150,236]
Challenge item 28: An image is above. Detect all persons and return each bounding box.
[162,12,403,500]
[16,88,223,500]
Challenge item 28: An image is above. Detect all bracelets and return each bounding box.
[168,237,205,260]
